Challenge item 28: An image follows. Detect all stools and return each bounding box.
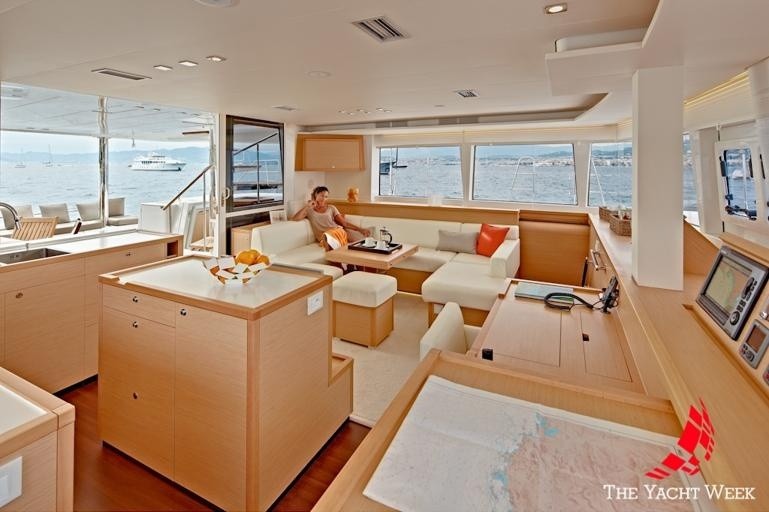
[332,271,397,347]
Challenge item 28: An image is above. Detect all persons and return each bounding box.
[293,186,371,271]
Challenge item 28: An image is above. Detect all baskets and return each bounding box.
[609,214,632,236]
[599,206,628,222]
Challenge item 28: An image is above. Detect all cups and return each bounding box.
[377,241,385,249]
[365,237,373,246]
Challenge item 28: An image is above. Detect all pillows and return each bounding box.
[0,204,32,230]
[39,203,70,223]
[76,204,98,220]
[109,198,125,216]
[435,230,480,254]
[477,223,510,256]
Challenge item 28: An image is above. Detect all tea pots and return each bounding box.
[380,226,392,248]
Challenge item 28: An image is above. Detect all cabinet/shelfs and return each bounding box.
[98,253,354,510]
[0,228,184,395]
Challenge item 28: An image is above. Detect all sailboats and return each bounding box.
[41,142,62,168]
[14,146,28,168]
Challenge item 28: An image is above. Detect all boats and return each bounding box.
[233,162,261,172]
[381,161,408,176]
[727,168,749,181]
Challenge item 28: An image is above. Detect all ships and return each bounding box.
[125,150,186,173]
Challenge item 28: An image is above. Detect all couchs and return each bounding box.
[304,214,463,294]
[419,301,482,361]
[251,219,343,279]
[422,223,521,325]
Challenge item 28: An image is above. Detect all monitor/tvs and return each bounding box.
[699,255,753,319]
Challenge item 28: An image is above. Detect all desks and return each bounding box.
[309,347,723,511]
[468,275,650,398]
[0,366,77,512]
